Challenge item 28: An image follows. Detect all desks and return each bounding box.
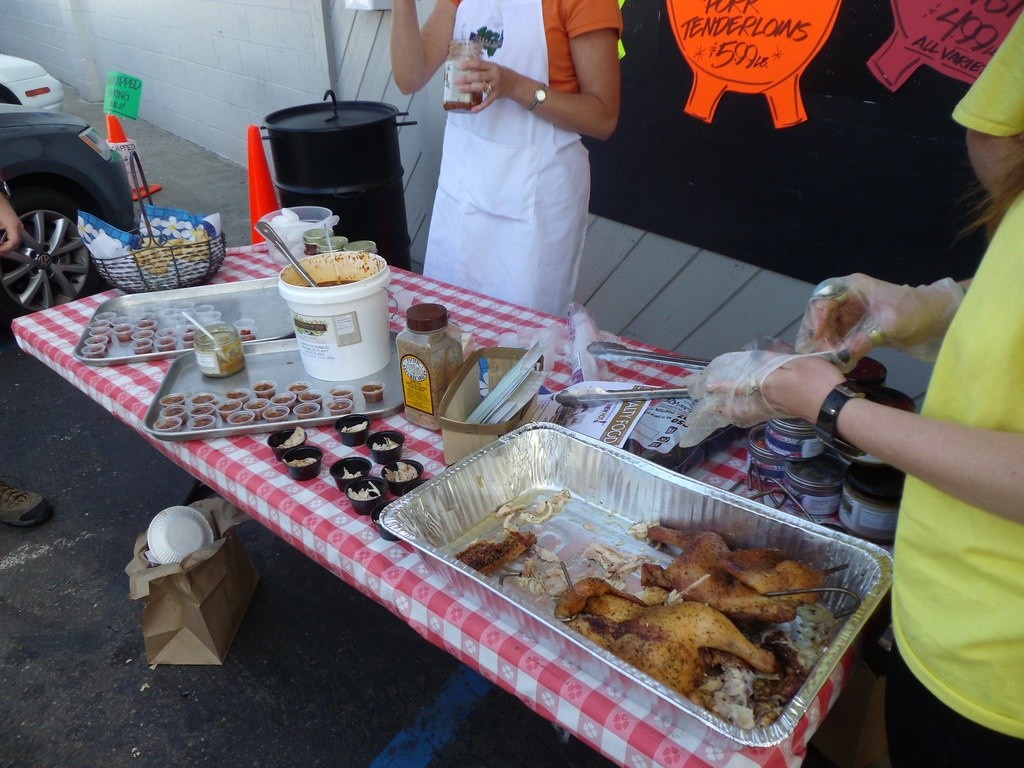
[11,242,894,768]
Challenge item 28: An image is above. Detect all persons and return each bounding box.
[0,192,54,527]
[392,0,622,321]
[704,9,1024,768]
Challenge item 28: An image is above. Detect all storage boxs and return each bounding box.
[437,347,544,465]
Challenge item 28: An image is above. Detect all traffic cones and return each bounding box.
[246,124,279,244]
[107,114,164,203]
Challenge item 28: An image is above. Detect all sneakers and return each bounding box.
[0,480,53,528]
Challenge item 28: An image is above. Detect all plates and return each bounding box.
[147,505,214,564]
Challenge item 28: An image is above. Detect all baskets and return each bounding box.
[89,150,226,295]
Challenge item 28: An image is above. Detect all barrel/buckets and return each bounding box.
[279,250,398,382]
[259,88,418,271]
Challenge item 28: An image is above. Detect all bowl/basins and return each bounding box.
[259,205,334,264]
[80,300,259,358]
[267,415,434,541]
[153,379,385,432]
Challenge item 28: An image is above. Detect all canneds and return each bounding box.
[748,356,917,545]
[193,324,245,378]
[302,227,378,257]
[442,39,484,113]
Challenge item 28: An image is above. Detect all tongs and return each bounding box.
[555,341,712,409]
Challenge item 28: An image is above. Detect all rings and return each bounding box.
[807,278,852,306]
[487,83,491,95]
[863,319,888,348]
[747,375,761,397]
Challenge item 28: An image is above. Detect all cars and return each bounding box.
[0,52,64,118]
[0,99,141,338]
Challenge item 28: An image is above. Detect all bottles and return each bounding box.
[343,240,378,256]
[192,322,245,378]
[302,228,334,255]
[316,236,348,255]
[394,303,463,431]
[443,39,485,114]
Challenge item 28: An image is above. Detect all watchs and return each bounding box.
[527,82,547,112]
[813,381,867,458]
[0,181,11,199]
